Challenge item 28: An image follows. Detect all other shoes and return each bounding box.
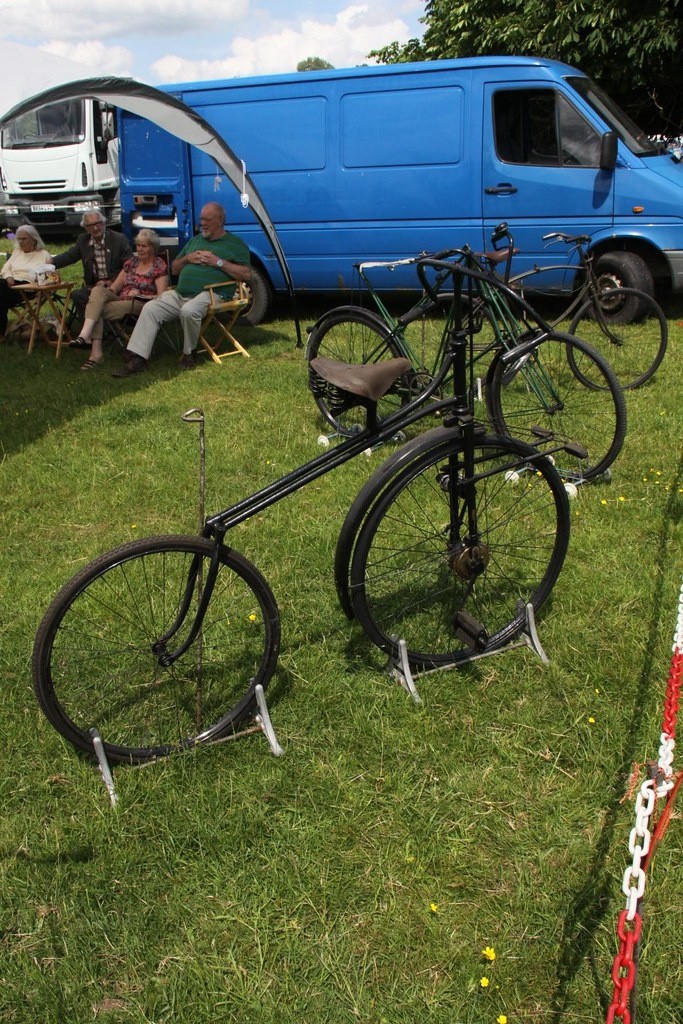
[177,350,198,370]
[0,334,5,344]
[111,356,146,377]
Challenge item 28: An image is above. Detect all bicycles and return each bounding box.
[31,258,573,768]
[305,219,668,481]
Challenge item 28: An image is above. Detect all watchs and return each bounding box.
[218,258,223,269]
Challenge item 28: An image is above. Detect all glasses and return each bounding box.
[85,221,103,229]
[16,236,31,241]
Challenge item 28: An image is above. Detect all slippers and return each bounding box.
[80,359,106,371]
[68,337,92,350]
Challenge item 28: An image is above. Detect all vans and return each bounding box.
[114,54,683,326]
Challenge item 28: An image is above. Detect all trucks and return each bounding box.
[0,96,119,238]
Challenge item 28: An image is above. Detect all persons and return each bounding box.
[46,210,134,327]
[66,228,169,374]
[0,224,51,340]
[111,202,252,377]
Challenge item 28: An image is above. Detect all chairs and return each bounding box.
[87,285,178,357]
[196,281,251,364]
[5,290,57,341]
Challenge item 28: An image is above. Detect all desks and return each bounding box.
[11,282,76,358]
